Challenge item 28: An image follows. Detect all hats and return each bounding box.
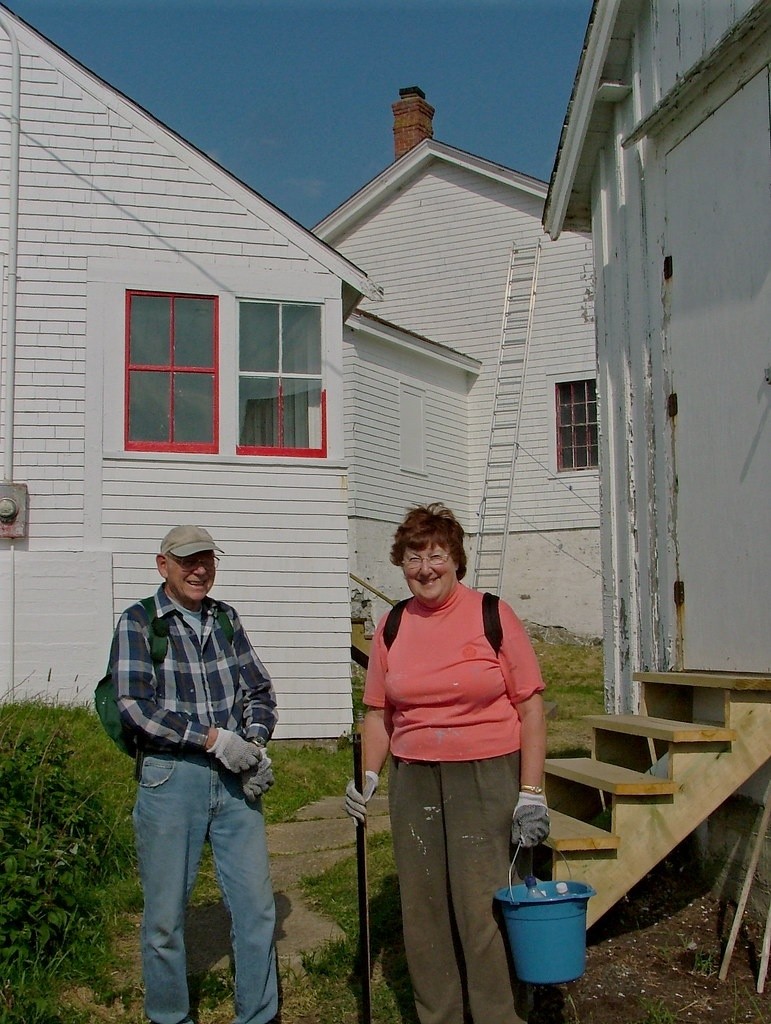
[160,525,225,557]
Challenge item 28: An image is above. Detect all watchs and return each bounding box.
[247,737,265,748]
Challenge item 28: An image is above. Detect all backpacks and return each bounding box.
[93,597,234,758]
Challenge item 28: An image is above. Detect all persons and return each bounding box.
[112,524,281,1024]
[344,500,549,1024]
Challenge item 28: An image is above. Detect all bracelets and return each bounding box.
[521,783,542,793]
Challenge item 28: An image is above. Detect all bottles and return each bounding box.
[555,882,570,896]
[525,876,545,899]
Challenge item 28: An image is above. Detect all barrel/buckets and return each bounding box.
[495,837,596,984]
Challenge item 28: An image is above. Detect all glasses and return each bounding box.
[167,553,220,570]
[400,553,451,568]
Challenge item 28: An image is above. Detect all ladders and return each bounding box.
[471,237,544,598]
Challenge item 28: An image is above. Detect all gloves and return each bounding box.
[510,792,552,849]
[206,727,262,773]
[344,770,379,827]
[240,740,275,803]
